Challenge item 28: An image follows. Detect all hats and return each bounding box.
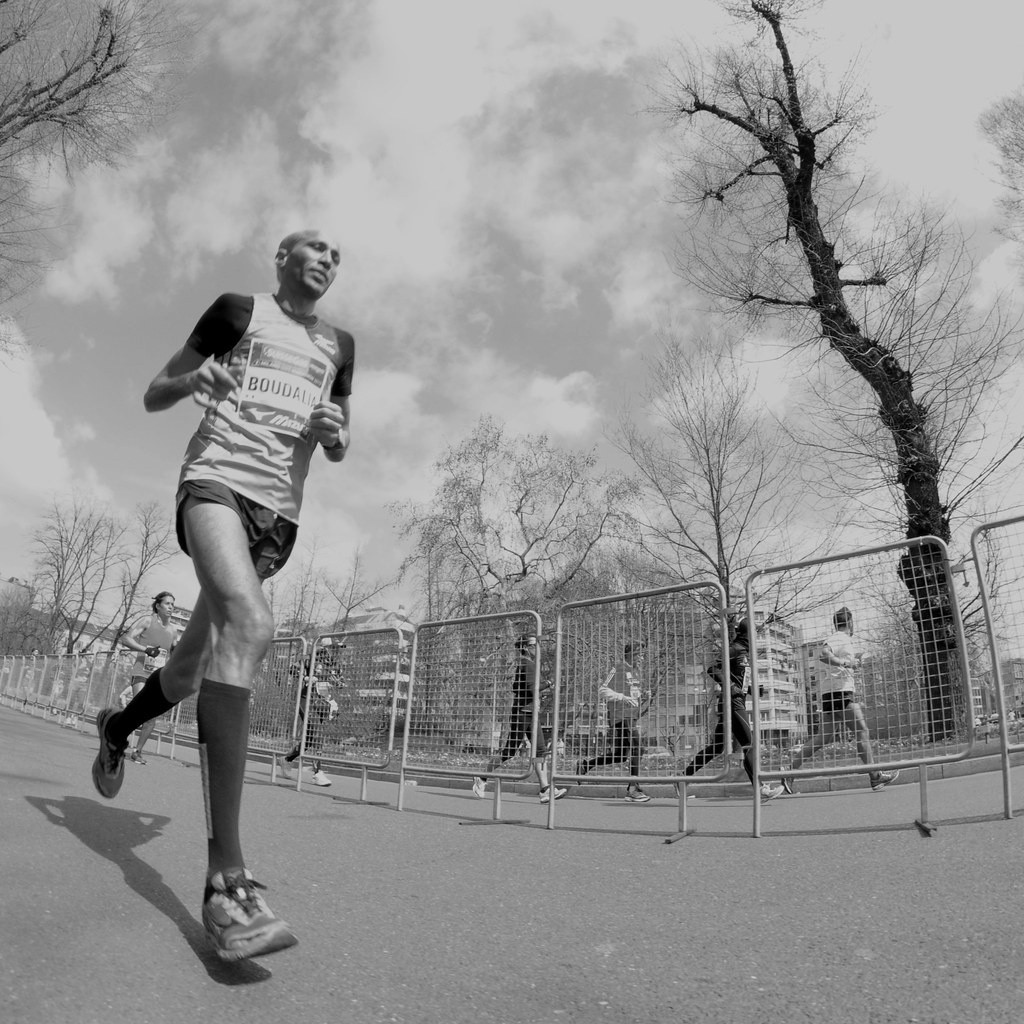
[321,637,347,649]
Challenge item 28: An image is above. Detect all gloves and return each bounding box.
[760,685,765,697]
[731,685,743,700]
[145,645,160,658]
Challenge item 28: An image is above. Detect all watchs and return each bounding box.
[322,429,347,452]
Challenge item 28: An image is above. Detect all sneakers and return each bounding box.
[760,784,784,804]
[203,865,301,964]
[671,772,680,798]
[871,770,899,790]
[311,770,331,786]
[471,776,486,798]
[278,757,295,779]
[779,765,794,794]
[576,759,588,785]
[130,750,147,765]
[92,707,129,800]
[625,789,650,802]
[539,787,568,804]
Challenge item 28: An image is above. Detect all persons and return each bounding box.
[672,617,784,804]
[117,685,133,709]
[66,648,91,725]
[278,636,347,787]
[0,657,13,693]
[49,656,68,715]
[472,633,568,804]
[576,642,652,802]
[92,228,356,963]
[778,606,901,794]
[121,591,177,765]
[23,648,42,712]
